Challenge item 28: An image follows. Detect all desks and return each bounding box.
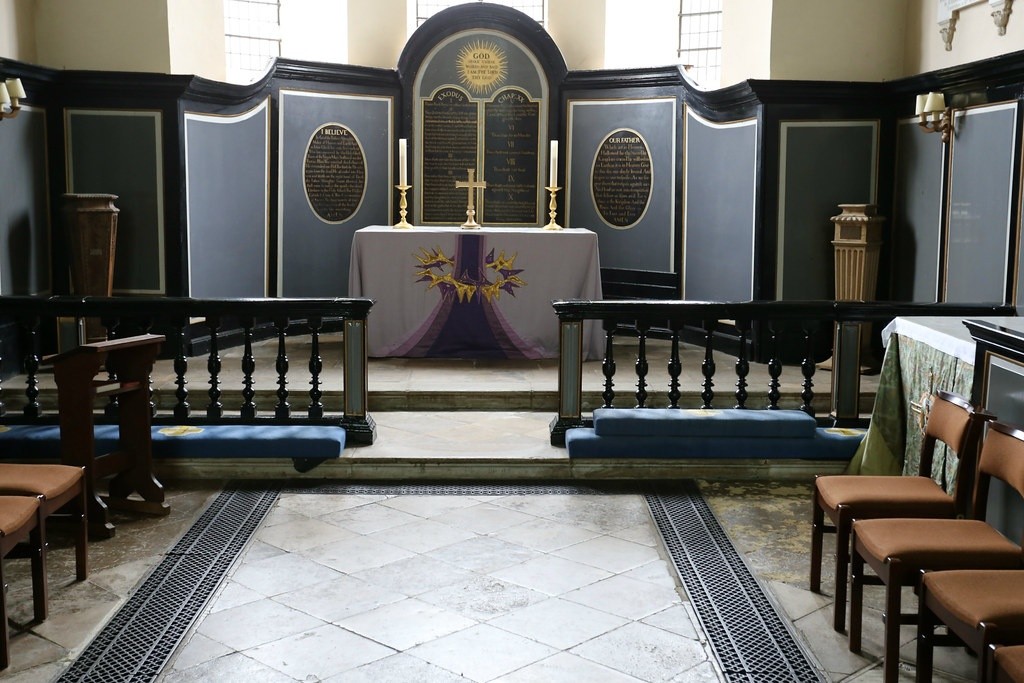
[347,225,606,360]
[845,317,1024,499]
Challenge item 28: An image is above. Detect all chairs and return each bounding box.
[912,569,1024,683]
[850,418,1024,683]
[810,388,996,631]
[986,643,1024,683]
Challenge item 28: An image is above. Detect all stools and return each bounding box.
[0,495,46,669]
[0,462,88,617]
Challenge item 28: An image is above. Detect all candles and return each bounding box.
[550,140,558,187]
[400,138,407,187]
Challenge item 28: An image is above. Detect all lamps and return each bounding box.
[915,91,951,144]
[0,78,26,121]
